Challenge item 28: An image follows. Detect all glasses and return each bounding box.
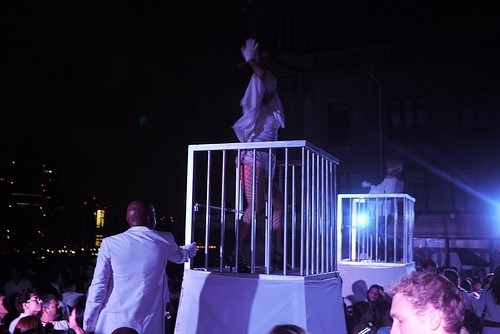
[25,297,41,302]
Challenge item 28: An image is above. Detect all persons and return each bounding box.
[82,199,197,334]
[0,250,500,334]
[222,38,293,273]
[361,158,406,262]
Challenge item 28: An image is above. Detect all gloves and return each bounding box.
[182,242,199,259]
[242,38,259,62]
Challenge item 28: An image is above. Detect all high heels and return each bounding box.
[226,254,249,274]
[272,251,292,272]
